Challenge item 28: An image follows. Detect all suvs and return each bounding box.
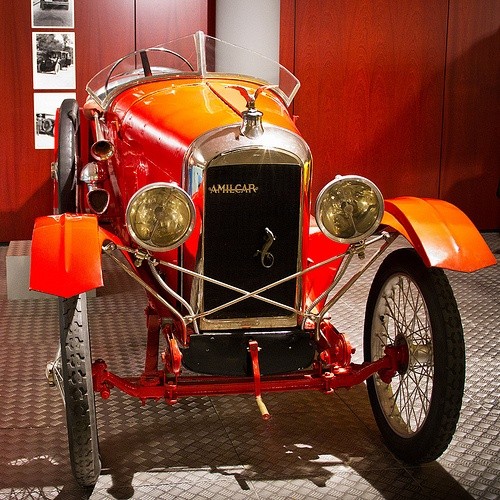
[37,49,71,74]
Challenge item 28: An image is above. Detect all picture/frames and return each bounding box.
[29,0,76,29]
[31,31,77,89]
[33,92,78,151]
